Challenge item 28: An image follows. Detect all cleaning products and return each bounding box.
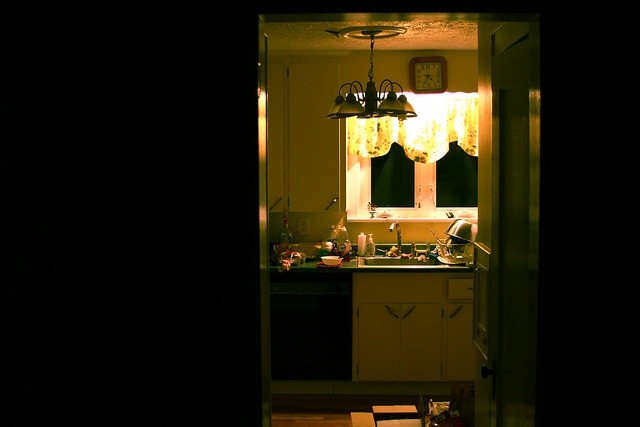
[337,225,352,257]
[357,256,443,265]
[325,225,339,256]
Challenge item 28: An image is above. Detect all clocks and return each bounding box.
[409,56,448,94]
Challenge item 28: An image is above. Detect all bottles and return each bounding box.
[279,216,292,250]
[328,224,338,238]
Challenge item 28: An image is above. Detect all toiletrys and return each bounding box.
[366,233,375,256]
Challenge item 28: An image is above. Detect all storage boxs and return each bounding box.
[350,394,425,427]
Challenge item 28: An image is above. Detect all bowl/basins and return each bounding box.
[321,255,344,265]
[444,219,478,243]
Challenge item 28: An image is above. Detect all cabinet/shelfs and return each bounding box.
[351,266,445,396]
[287,61,340,213]
[447,266,476,396]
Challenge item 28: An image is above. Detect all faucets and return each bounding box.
[389,221,402,255]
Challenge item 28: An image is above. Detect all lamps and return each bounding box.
[326,25,417,122]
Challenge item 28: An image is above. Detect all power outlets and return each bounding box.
[296,218,311,236]
